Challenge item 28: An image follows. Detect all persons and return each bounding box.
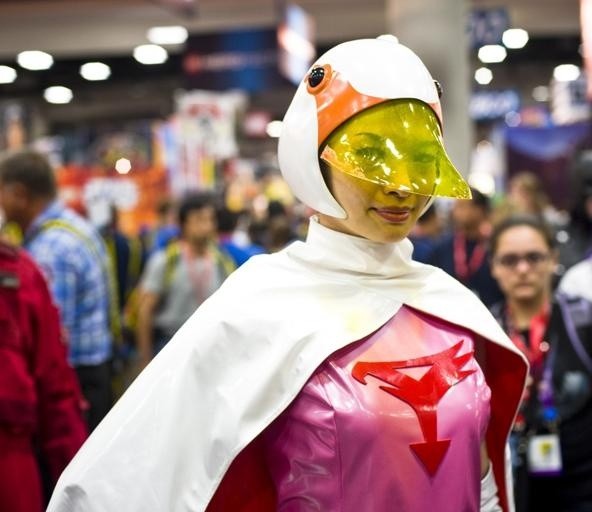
[0,153,117,424]
[415,174,573,306]
[43,34,530,510]
[0,242,90,512]
[484,212,592,512]
[100,190,309,373]
[555,174,592,300]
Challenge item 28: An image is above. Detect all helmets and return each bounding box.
[276,30,449,222]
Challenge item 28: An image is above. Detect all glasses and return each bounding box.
[490,252,556,271]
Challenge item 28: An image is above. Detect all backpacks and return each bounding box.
[120,281,145,332]
